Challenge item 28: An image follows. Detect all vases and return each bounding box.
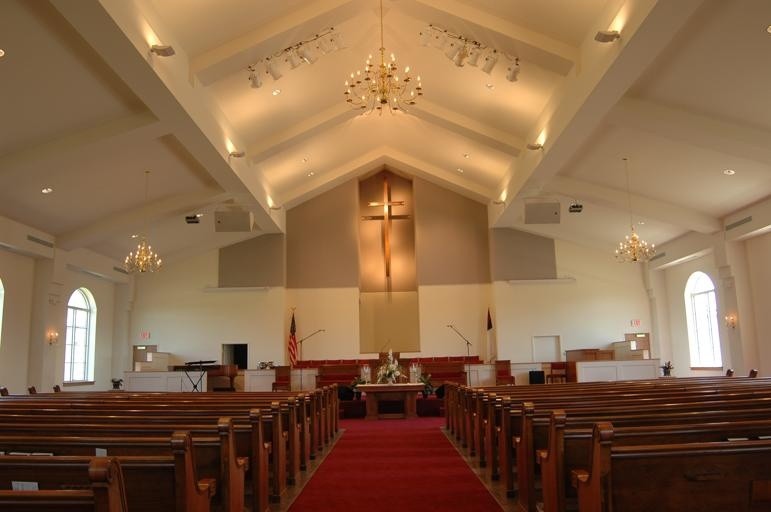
[385,377,395,384]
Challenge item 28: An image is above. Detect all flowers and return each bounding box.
[375,349,409,383]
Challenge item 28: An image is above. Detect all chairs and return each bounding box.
[272,366,291,393]
[495,360,515,386]
[546,361,568,384]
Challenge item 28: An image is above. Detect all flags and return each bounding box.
[288,313,297,369]
[486,308,496,363]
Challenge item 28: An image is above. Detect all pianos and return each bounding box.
[168,361,238,392]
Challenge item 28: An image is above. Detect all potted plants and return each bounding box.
[349,376,367,400]
[418,375,438,399]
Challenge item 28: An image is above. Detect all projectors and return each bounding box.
[569,205,582,213]
[185,215,200,224]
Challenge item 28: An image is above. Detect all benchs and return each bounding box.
[0,383,340,512]
[443,370,771,512]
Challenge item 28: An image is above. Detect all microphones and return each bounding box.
[317,328,327,332]
[446,324,453,328]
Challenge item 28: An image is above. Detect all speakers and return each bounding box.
[524,201,561,226]
[214,210,255,232]
[528,371,545,383]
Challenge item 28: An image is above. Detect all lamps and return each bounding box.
[151,45,175,57]
[613,157,657,265]
[344,0,424,117]
[527,144,543,150]
[124,169,164,273]
[724,313,737,329]
[420,23,521,83]
[247,26,348,88]
[269,205,281,210]
[230,151,245,157]
[49,333,59,345]
[594,31,620,42]
[493,199,505,204]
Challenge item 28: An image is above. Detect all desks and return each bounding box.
[355,383,426,421]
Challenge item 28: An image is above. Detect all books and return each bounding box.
[31,452,53,457]
[9,452,31,456]
[758,436,771,440]
[11,480,40,491]
[95,448,108,457]
[728,437,750,441]
